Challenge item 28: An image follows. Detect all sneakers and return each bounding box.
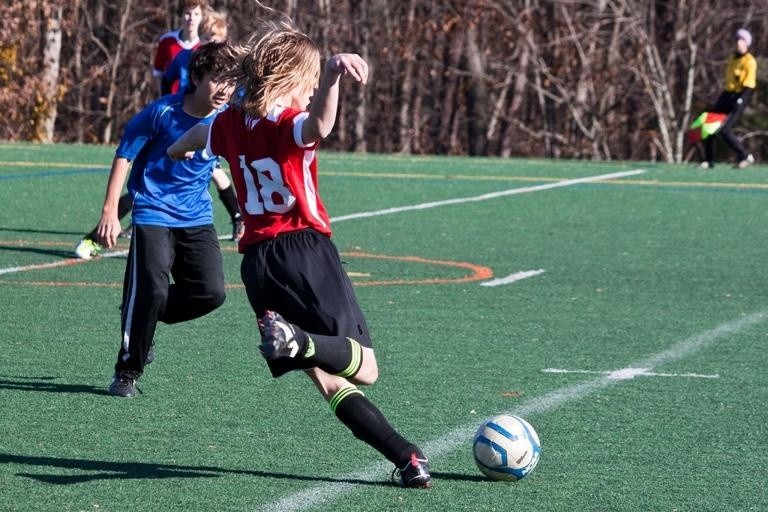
[109,337,155,398]
[75,232,100,259]
[258,310,303,360]
[392,447,431,488]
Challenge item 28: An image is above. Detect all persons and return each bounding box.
[153,1,245,242]
[75,11,228,260]
[97,43,238,396]
[698,28,757,169]
[166,0,430,489]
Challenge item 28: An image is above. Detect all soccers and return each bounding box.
[472,413,540,481]
